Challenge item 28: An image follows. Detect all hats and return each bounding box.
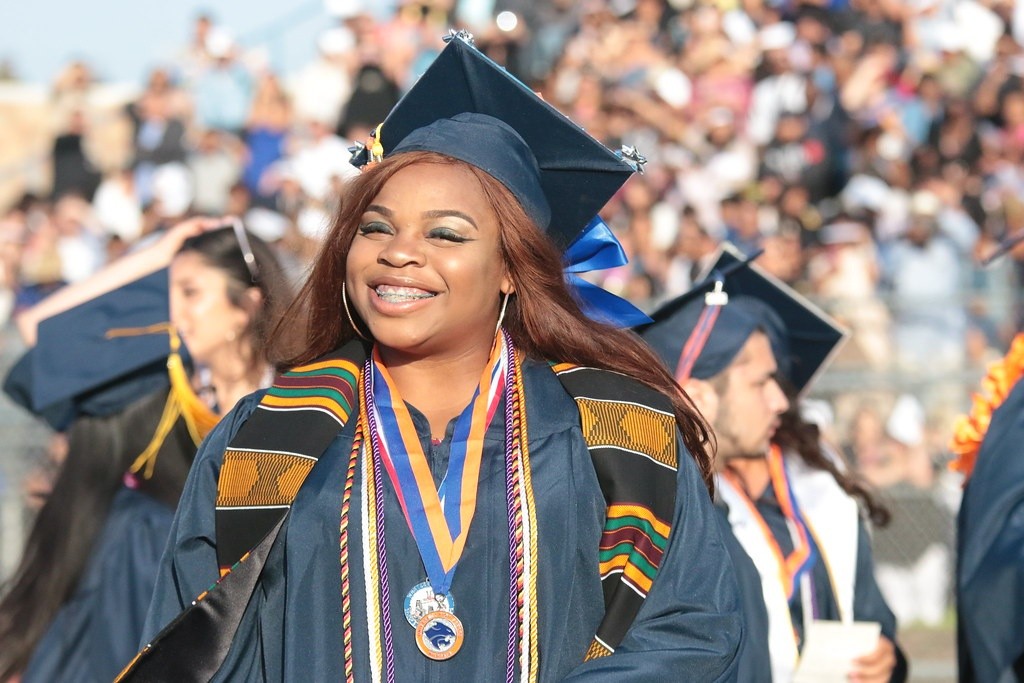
[348,27,646,248]
[205,33,234,58]
[698,244,845,395]
[303,106,338,126]
[626,248,768,391]
[33,265,223,476]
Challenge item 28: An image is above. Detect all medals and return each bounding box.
[415,609,464,661]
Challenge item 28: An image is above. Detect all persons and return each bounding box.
[0,0,1024,683]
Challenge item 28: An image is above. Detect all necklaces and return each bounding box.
[339,323,538,682]
